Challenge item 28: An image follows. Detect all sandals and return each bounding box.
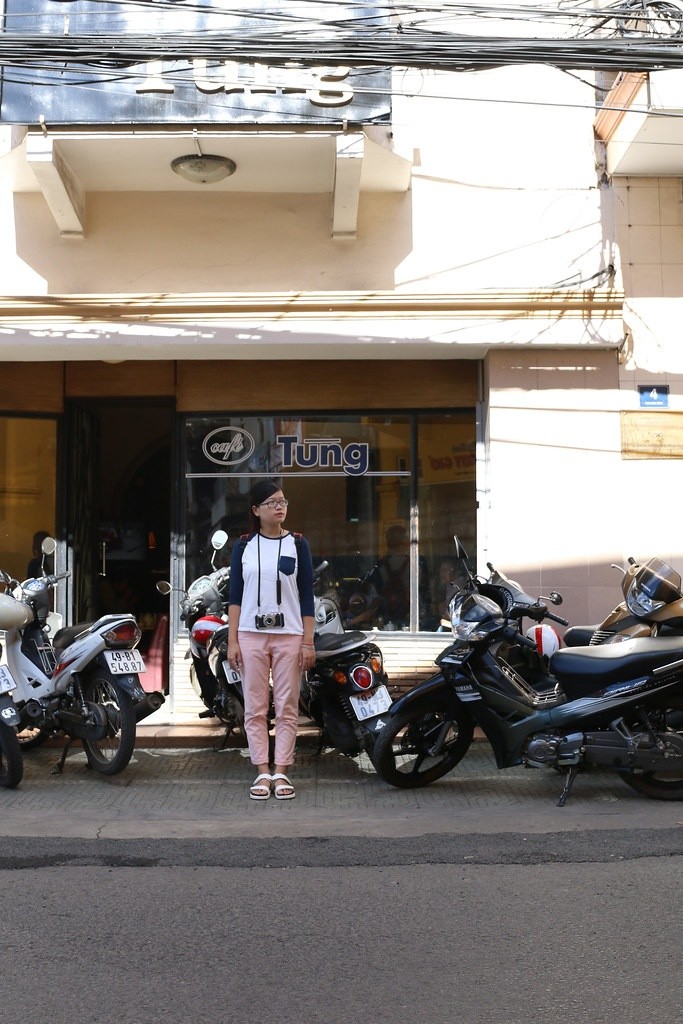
[272,773,296,799]
[250,774,273,799]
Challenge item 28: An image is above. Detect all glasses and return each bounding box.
[258,499,288,508]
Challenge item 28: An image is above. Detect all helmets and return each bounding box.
[190,616,227,659]
[527,624,561,658]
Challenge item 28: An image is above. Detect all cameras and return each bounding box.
[255,613,285,629]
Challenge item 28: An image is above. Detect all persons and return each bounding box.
[438,555,467,632]
[228,480,317,800]
[372,526,431,632]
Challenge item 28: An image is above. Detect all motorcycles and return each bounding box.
[372,535,683,807]
[0,537,165,776]
[155,529,275,750]
[589,557,683,648]
[337,566,386,631]
[482,563,603,704]
[0,645,25,789]
[296,560,399,780]
